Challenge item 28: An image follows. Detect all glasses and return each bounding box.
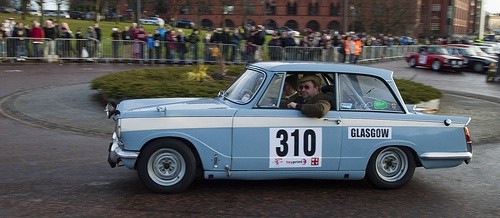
[299,85,309,89]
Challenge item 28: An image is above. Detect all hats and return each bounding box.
[285,75,299,91]
[297,75,321,86]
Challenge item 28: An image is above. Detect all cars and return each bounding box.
[139,16,165,27]
[0,6,106,21]
[405,46,468,71]
[105,60,472,188]
[469,42,500,60]
[449,48,499,71]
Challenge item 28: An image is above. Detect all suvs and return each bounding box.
[171,19,194,29]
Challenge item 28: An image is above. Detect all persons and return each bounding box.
[279,72,335,119]
[273,74,303,108]
[0,16,482,65]
